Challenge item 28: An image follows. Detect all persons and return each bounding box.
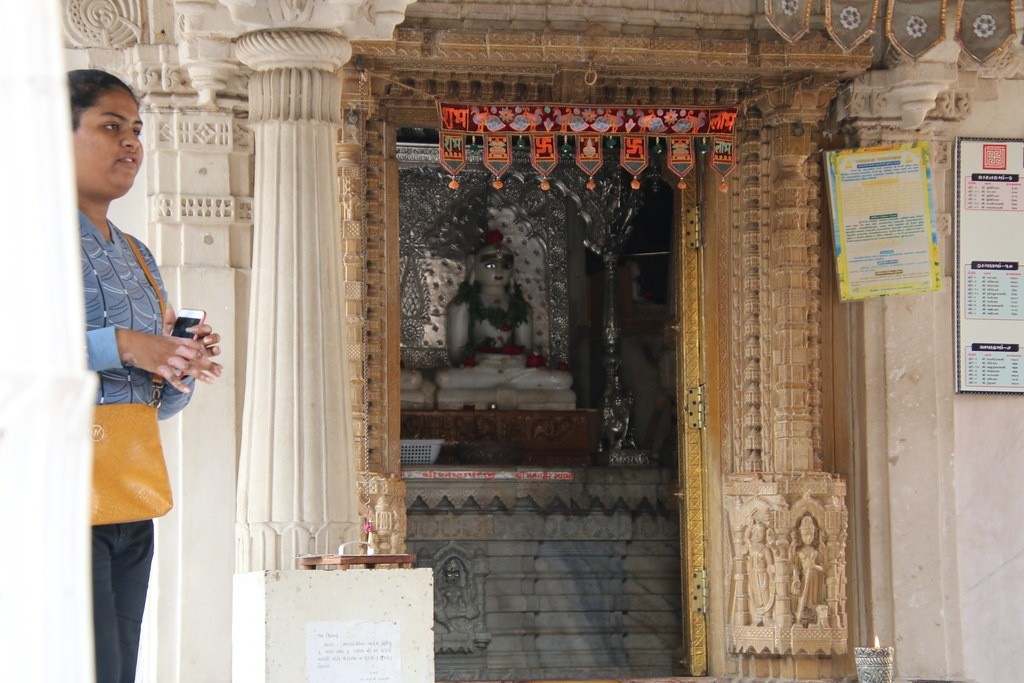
[66,69,220,682]
[435,235,573,388]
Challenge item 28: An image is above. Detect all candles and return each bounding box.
[854,636,894,683]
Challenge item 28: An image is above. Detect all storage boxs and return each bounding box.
[400,439,445,464]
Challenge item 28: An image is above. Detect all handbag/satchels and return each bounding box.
[89,403,173,525]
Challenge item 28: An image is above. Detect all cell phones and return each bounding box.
[171,309,207,380]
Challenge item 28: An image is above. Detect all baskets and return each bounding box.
[400,439,445,465]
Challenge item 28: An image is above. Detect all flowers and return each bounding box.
[460,229,547,369]
